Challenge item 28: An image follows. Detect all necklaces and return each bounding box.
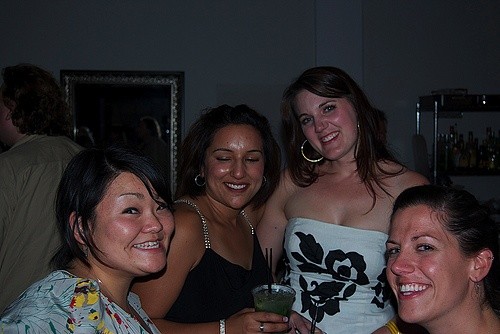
[95,275,136,320]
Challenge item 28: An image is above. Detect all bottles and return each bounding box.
[446,126,500,176]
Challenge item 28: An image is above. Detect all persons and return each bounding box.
[0,146,174,334]
[386,182,500,334]
[0,64,85,324]
[126,104,323,334]
[260,65,431,334]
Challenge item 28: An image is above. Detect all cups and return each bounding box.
[252,283,297,331]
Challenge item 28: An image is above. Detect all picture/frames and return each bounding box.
[60,70,185,201]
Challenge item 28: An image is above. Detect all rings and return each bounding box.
[260,322,264,331]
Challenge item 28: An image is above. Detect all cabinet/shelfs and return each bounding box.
[416,94,500,185]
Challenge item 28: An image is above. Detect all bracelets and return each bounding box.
[384,320,402,334]
[220,318,225,334]
